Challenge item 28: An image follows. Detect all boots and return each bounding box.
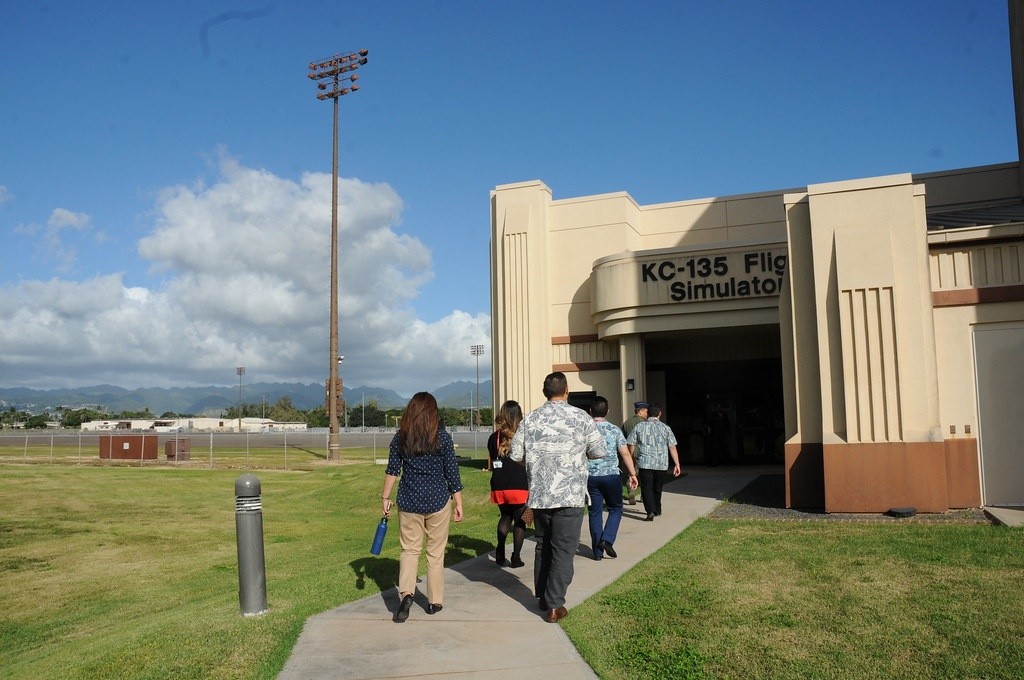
[511,552,524,568]
[496,548,505,566]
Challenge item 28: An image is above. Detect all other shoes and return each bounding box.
[645,512,655,521]
[655,513,661,516]
[629,493,636,505]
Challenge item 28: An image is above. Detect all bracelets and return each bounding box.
[381,496,389,499]
[630,474,637,476]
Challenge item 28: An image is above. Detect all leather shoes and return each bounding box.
[547,607,568,623]
[600,541,617,557]
[539,599,548,611]
[397,594,413,623]
[428,604,442,614]
[594,555,605,560]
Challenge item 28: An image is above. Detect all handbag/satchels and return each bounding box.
[521,508,533,527]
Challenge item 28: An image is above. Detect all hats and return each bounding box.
[634,402,649,408]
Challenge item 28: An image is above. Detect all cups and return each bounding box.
[370,517,388,555]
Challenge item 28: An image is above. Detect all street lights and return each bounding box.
[236,366,246,430]
[306,47,370,458]
[470,345,485,428]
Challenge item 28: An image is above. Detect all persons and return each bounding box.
[626,405,681,521]
[381,392,463,623]
[702,402,733,467]
[620,401,649,504]
[486,401,529,568]
[509,372,607,623]
[585,396,638,561]
